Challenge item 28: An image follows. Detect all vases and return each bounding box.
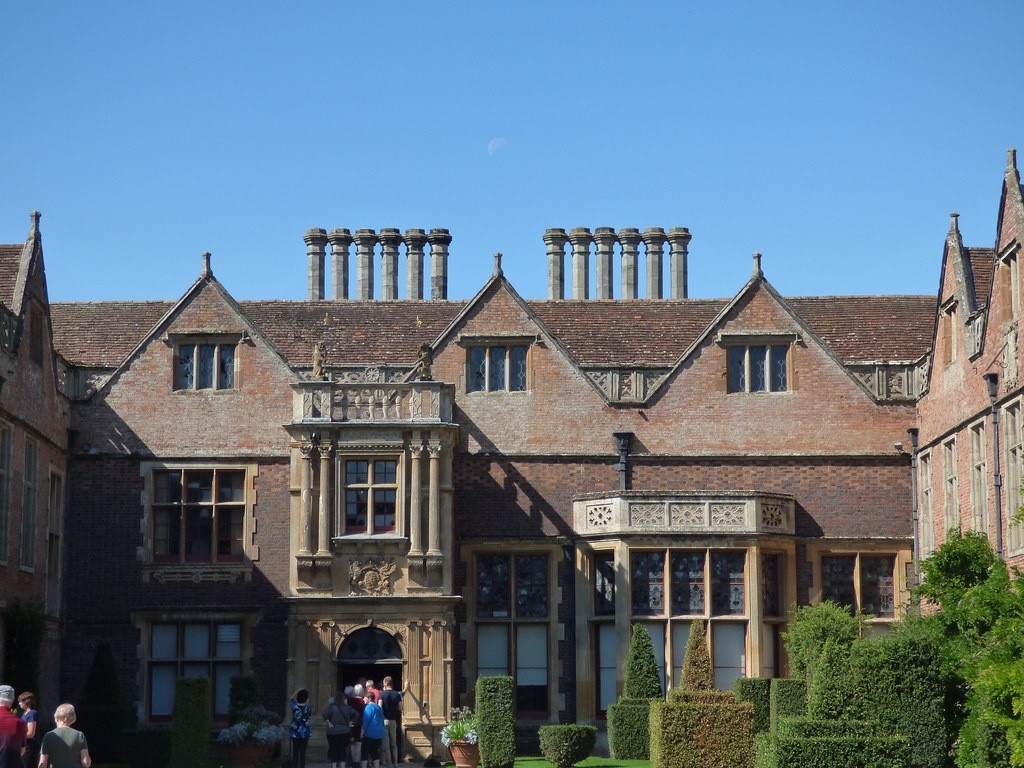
[223,742,275,768]
[449,740,481,768]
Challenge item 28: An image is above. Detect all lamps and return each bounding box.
[894,442,902,451]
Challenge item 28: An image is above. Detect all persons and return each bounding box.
[288,689,312,768]
[344,676,409,768]
[322,693,360,768]
[360,691,385,768]
[38,703,92,768]
[0,684,28,768]
[18,691,38,768]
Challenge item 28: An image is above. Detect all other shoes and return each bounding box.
[367,762,372,768]
[385,764,399,768]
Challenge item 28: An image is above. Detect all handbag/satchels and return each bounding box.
[349,731,355,744]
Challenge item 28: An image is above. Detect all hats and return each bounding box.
[0,685,14,699]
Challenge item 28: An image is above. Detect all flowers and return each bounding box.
[439,705,478,748]
[215,721,285,747]
